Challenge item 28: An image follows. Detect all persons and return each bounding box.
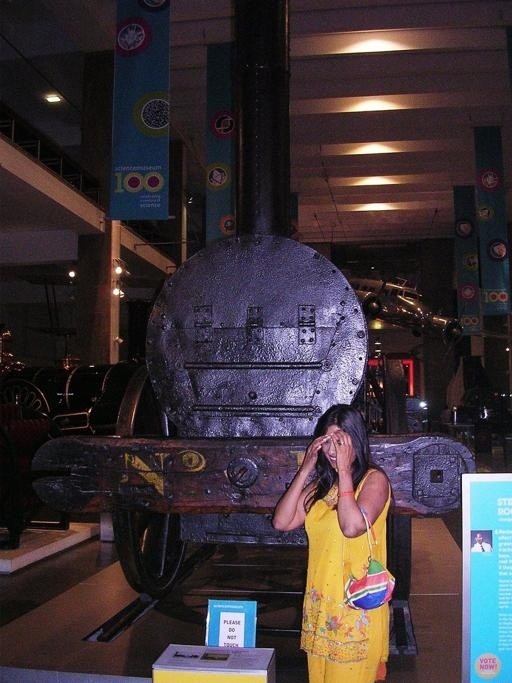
[470,532,492,552]
[272,404,390,683]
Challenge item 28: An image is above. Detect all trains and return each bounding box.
[29,235,473,658]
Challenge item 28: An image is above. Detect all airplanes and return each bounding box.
[345,273,512,349]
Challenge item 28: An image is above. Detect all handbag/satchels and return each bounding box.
[343,561,396,610]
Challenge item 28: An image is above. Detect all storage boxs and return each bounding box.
[150,643,276,683]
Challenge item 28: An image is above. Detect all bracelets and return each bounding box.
[338,491,355,497]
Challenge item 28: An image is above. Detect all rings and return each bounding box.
[341,442,345,445]
